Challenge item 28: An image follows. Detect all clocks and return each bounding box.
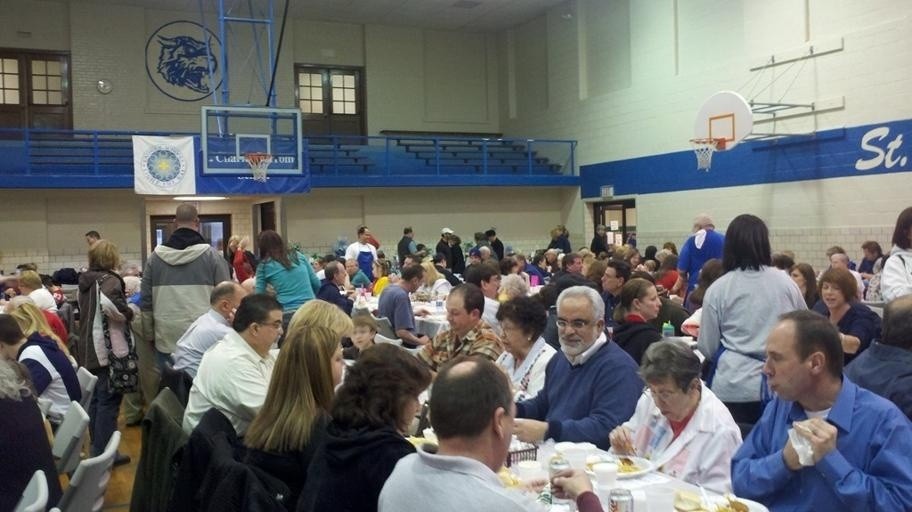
[96,79,113,94]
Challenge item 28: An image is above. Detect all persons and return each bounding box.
[78,240,140,467]
[122,204,320,428]
[378,355,603,511]
[85,231,101,246]
[313,224,689,402]
[512,287,646,452]
[843,308,911,420]
[1,262,80,511]
[772,204,911,368]
[245,326,348,496]
[697,213,809,424]
[607,340,743,497]
[303,343,433,510]
[672,213,725,305]
[181,293,284,437]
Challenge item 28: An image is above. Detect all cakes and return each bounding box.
[549,456,571,500]
[608,487,634,512]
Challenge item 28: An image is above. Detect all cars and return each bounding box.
[530,275,538,287]
[344,286,372,304]
[662,325,675,338]
[672,490,701,512]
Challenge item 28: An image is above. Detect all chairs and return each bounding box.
[351,294,449,339]
[91,446,132,465]
[49,431,121,512]
[50,401,90,477]
[76,367,98,412]
[151,387,266,509]
[13,470,49,512]
[58,287,80,360]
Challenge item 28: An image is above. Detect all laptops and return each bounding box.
[672,489,701,512]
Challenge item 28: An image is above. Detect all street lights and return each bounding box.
[105,351,140,395]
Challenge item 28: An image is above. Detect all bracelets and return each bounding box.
[544,431,549,442]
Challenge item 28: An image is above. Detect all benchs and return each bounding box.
[1,129,564,177]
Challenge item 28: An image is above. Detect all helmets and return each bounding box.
[584,455,657,477]
[707,497,772,511]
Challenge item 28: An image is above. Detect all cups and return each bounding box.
[640,374,695,401]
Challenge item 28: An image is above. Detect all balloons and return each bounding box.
[96,79,113,94]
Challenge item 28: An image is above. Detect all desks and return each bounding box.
[513,439,768,510]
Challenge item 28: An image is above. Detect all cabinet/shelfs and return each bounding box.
[411,291,448,315]
[548,446,572,511]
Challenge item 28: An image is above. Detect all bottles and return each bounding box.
[440,227,454,235]
[468,246,480,259]
